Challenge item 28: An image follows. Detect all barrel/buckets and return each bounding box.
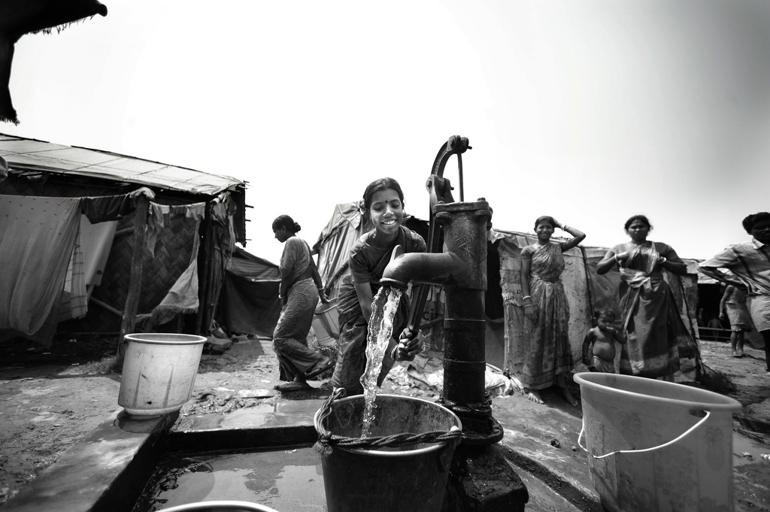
[571,371,743,512]
[313,388,463,512]
[118,332,207,419]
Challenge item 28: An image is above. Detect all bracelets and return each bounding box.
[390,344,398,361]
[656,256,668,266]
[319,287,325,297]
[277,294,286,300]
[523,295,533,301]
[615,252,618,263]
[560,224,567,231]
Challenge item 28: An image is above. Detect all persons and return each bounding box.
[271,215,332,393]
[519,214,587,410]
[332,176,429,400]
[696,306,707,339]
[418,285,445,360]
[718,284,754,357]
[579,306,628,374]
[708,313,724,341]
[596,213,689,379]
[695,210,769,374]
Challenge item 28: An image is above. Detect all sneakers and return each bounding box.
[273,381,308,392]
[304,356,335,380]
